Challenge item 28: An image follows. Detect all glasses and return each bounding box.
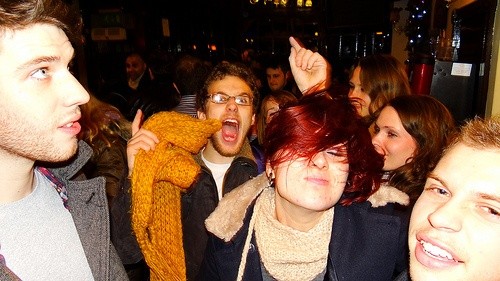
[205,93,252,106]
